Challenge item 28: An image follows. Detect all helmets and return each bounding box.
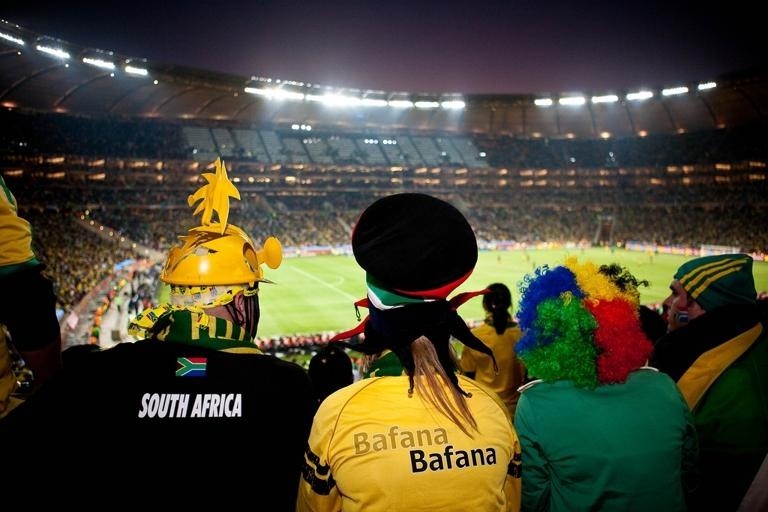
[159,223,277,286]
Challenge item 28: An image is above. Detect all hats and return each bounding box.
[673,254,757,315]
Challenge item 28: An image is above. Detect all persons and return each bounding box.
[1,158,323,511]
[293,191,522,511]
[1,145,177,315]
[254,326,364,385]
[307,339,355,405]
[514,256,697,511]
[462,284,530,418]
[654,254,767,510]
[174,145,768,259]
[0,178,63,420]
